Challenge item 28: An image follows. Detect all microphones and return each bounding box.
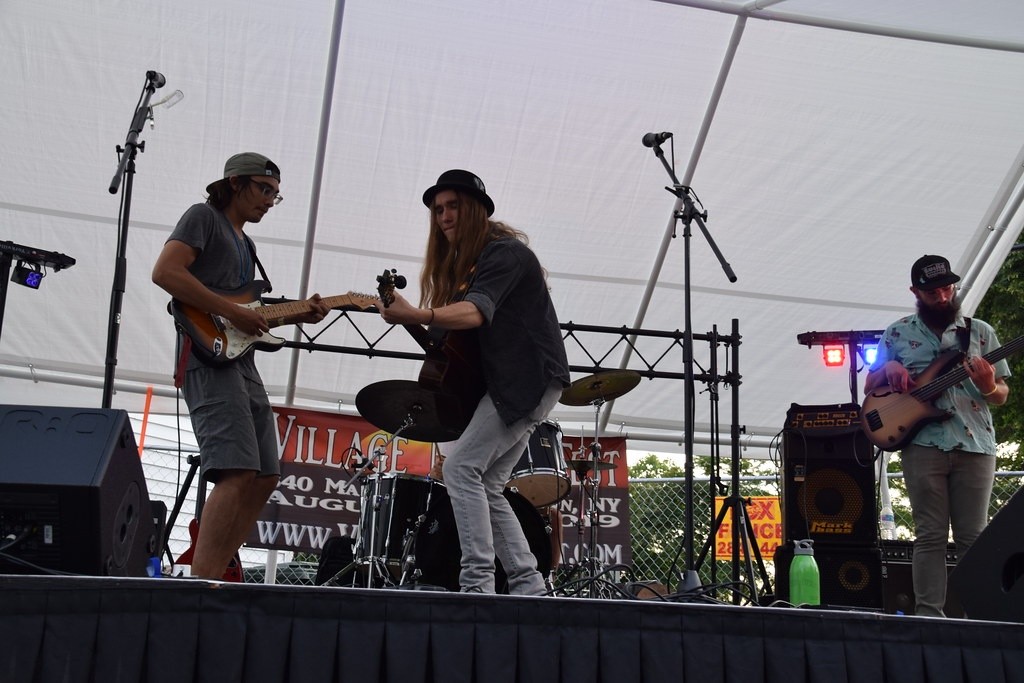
[642,132,673,147]
[147,70,166,88]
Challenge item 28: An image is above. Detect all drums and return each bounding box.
[411,486,553,594]
[355,472,447,566]
[504,419,571,508]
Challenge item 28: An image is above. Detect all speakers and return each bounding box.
[780,424,879,548]
[0,403,159,580]
[950,485,1024,624]
[773,545,884,612]
[881,560,956,618]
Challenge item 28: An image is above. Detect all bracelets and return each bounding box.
[421,308,434,325]
[980,384,997,396]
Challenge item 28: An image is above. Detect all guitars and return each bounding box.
[377,268,481,397]
[860,335,1024,451]
[171,279,382,365]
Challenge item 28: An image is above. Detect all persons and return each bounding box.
[151,152,331,581]
[372,169,571,595]
[865,254,1012,618]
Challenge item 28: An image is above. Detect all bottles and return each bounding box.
[789,538,821,609]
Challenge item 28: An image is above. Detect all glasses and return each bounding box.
[249,179,283,205]
[916,284,955,299]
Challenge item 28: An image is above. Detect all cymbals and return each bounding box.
[356,380,477,442]
[565,460,618,471]
[559,369,642,406]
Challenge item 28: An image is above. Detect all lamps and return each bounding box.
[10,260,48,290]
[861,345,878,365]
[822,348,845,367]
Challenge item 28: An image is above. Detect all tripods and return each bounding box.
[553,392,631,601]
[322,411,416,589]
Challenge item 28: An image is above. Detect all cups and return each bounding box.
[173,564,191,577]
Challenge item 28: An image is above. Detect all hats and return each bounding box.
[422,169,495,218]
[911,255,961,291]
[206,152,281,194]
